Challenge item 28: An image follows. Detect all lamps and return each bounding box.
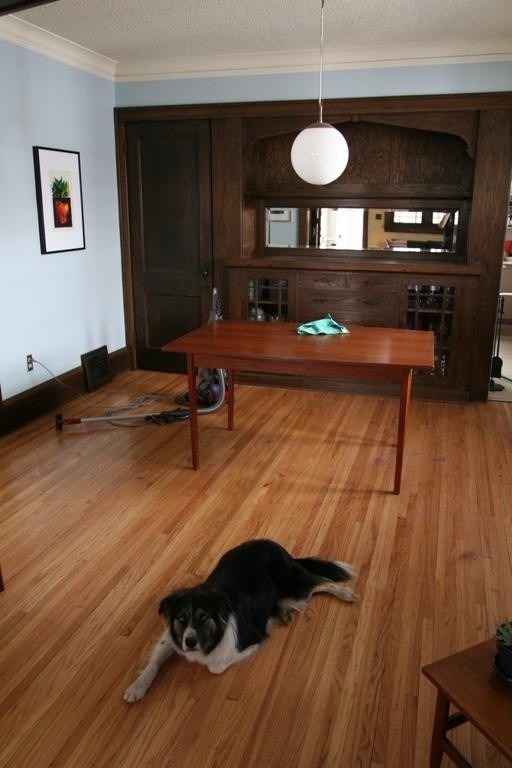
[290,0,349,187]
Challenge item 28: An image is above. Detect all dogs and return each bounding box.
[122,540,360,704]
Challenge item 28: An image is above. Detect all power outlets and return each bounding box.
[27,354,33,371]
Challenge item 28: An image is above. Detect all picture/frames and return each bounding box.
[32,146,84,254]
[269,207,291,222]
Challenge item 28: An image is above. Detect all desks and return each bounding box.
[161,319,435,494]
[422,638,512,768]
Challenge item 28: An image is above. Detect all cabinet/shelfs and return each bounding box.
[227,268,501,402]
[124,120,213,374]
